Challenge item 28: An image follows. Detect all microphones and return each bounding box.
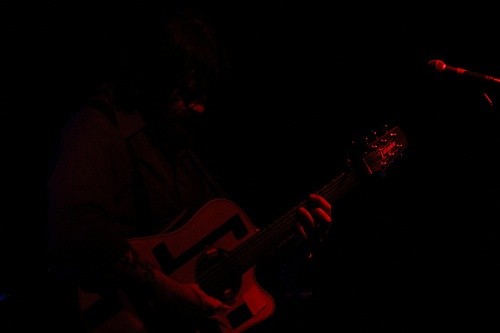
[428,60,489,81]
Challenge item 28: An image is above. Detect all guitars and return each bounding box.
[78,123,409,333]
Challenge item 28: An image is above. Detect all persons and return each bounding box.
[46,19,334,331]
